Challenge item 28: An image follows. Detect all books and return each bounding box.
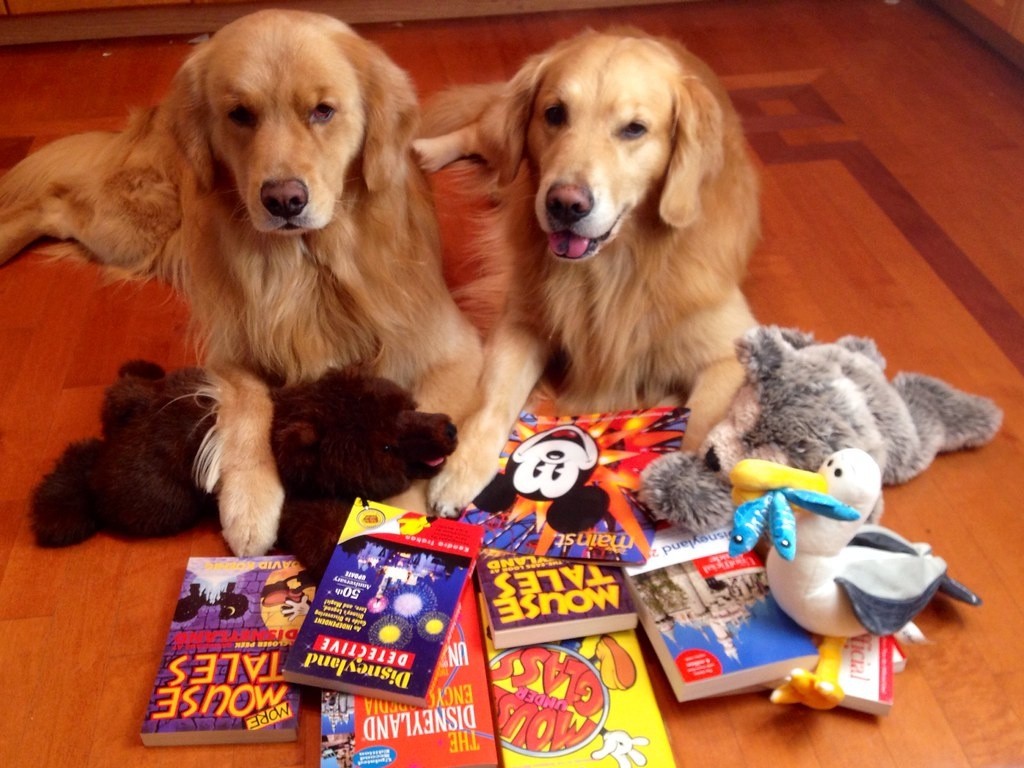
[141,552,317,746]
[281,370,905,768]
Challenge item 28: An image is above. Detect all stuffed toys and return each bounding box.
[637,323,1009,709]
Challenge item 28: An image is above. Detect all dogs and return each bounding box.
[0,8,764,558]
